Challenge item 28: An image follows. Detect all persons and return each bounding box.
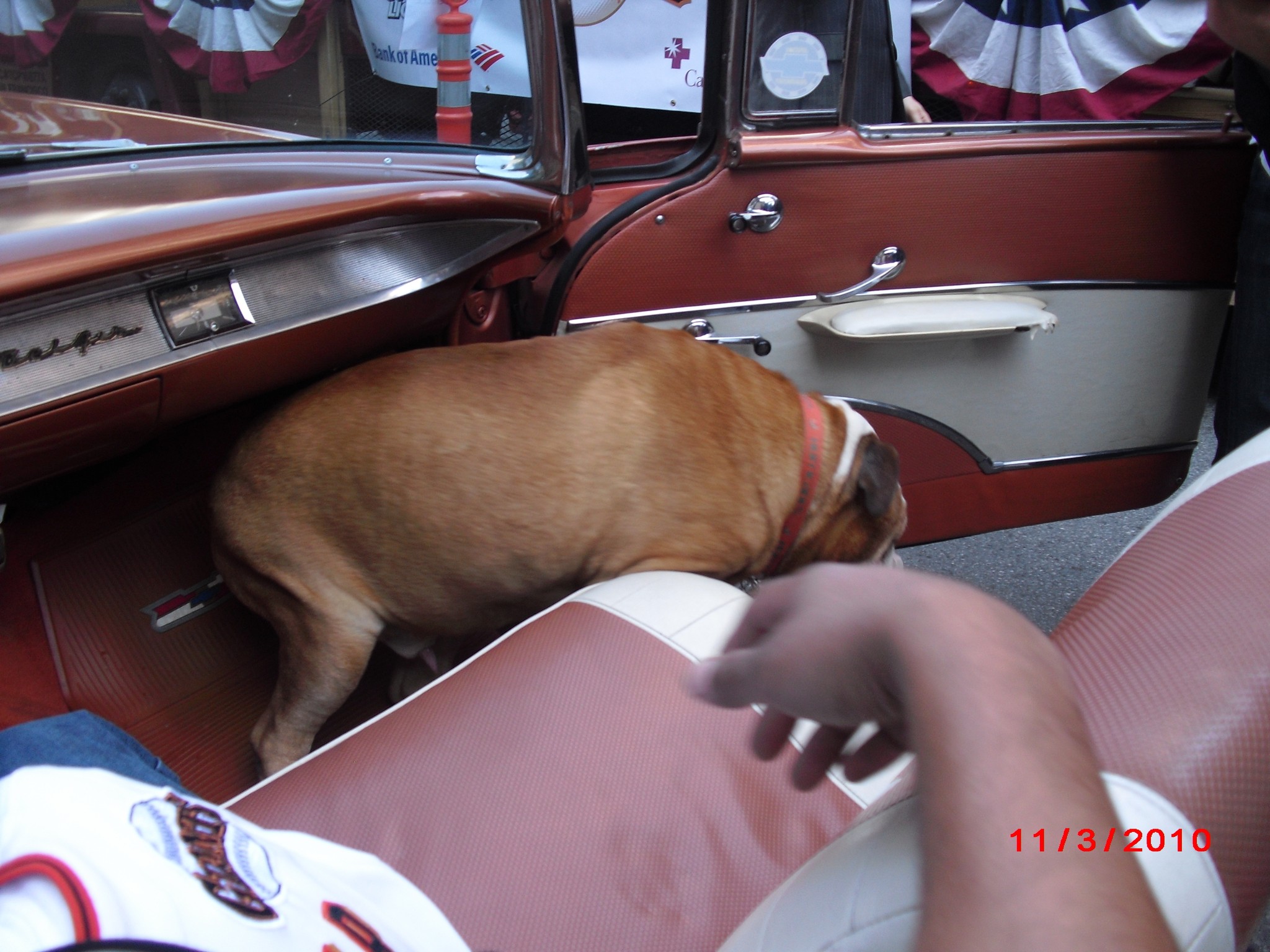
[746,0,933,127]
[685,563,1183,952]
[1205,0,1270,461]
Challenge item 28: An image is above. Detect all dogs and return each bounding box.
[204,323,907,781]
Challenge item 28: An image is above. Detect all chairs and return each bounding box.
[223,430,1270,952]
